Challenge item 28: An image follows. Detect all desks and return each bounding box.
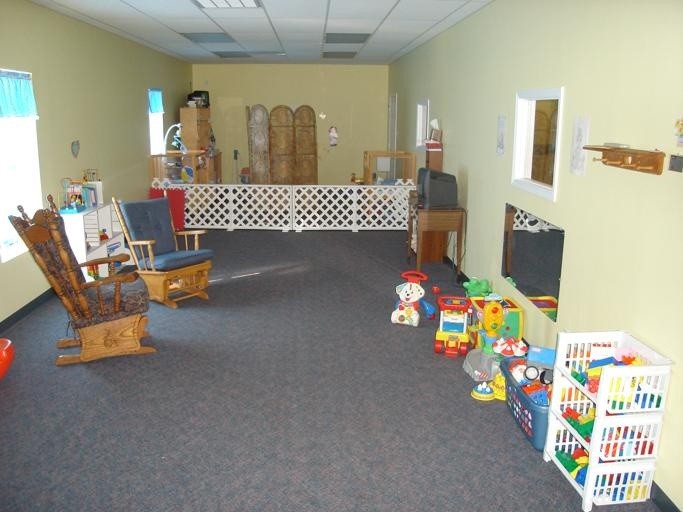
[406,190,464,278]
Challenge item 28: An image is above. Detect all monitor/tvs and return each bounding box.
[416,167,457,209]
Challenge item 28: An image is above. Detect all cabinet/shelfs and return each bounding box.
[180,108,221,202]
[542,330,676,512]
[60,199,136,282]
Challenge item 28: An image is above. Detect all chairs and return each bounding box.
[112,190,215,308]
[7,194,157,366]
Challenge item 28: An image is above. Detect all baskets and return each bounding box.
[500,356,548,451]
